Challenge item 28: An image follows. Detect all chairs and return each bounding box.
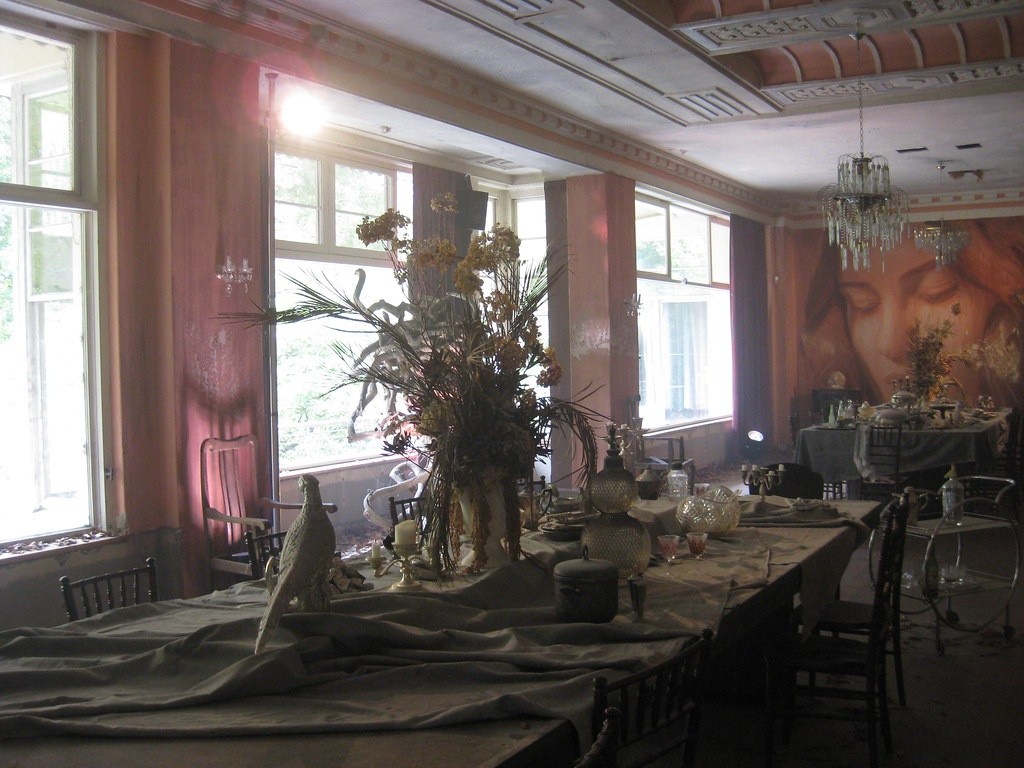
[621,394,696,496]
[788,412,842,499]
[200,435,337,592]
[809,388,862,498]
[763,491,904,768]
[853,423,908,498]
[389,496,427,534]
[959,406,1024,509]
[60,558,158,623]
[572,628,714,768]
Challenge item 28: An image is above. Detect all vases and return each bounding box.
[454,482,518,570]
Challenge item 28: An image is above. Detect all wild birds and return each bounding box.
[254,472,337,655]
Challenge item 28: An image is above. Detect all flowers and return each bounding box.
[894,313,976,404]
[210,192,616,591]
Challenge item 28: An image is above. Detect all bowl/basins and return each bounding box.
[536,523,582,540]
[858,407,874,416]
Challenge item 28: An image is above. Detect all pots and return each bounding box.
[552,545,620,626]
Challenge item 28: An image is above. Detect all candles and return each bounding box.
[742,465,748,471]
[778,464,785,471]
[752,464,758,471]
[769,470,775,474]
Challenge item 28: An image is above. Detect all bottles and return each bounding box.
[667,462,687,502]
[940,463,965,524]
[828,399,855,428]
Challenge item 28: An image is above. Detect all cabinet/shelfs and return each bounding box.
[867,476,1020,655]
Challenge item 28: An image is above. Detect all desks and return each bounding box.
[0,500,882,768]
[794,406,1014,501]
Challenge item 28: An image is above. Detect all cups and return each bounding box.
[686,532,708,558]
[938,562,967,583]
[657,534,679,562]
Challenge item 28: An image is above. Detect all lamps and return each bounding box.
[216,247,256,296]
[817,32,909,272]
[623,294,643,318]
[913,165,969,273]
